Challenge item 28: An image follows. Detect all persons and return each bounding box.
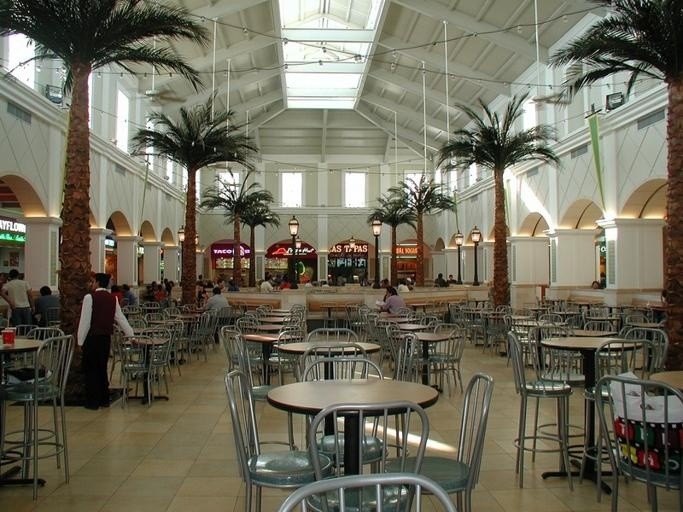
[207,287,230,314]
[447,274,457,286]
[197,274,208,288]
[78,274,134,409]
[29,285,62,326]
[197,284,206,299]
[0,269,35,334]
[306,280,312,287]
[398,279,410,291]
[150,281,159,291]
[280,277,292,291]
[260,275,274,293]
[156,284,165,302]
[228,280,240,291]
[361,277,379,290]
[381,287,407,315]
[322,281,329,287]
[144,286,155,300]
[110,285,124,302]
[121,285,138,307]
[214,279,230,292]
[163,278,169,289]
[435,273,449,287]
[591,281,600,289]
[600,272,606,289]
[382,279,391,288]
[166,282,172,296]
[201,291,210,301]
[169,281,174,288]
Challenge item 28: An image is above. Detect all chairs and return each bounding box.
[321,301,341,338]
[123,305,142,312]
[151,328,173,396]
[392,335,424,383]
[529,324,588,464]
[0,333,77,505]
[584,321,614,332]
[300,342,367,380]
[538,314,562,324]
[379,373,494,511]
[0,323,69,476]
[428,334,465,393]
[434,323,460,334]
[222,368,336,509]
[594,374,682,512]
[170,320,184,376]
[221,325,262,386]
[43,304,60,347]
[165,307,181,315]
[277,470,462,511]
[307,328,358,343]
[626,314,650,324]
[302,358,390,469]
[109,323,123,383]
[305,400,431,511]
[217,306,234,329]
[143,302,159,307]
[480,311,508,355]
[12,323,42,365]
[584,338,657,505]
[345,302,368,341]
[622,326,669,373]
[505,330,576,493]
[144,313,165,320]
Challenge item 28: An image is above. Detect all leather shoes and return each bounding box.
[84,401,102,410]
[99,398,112,407]
